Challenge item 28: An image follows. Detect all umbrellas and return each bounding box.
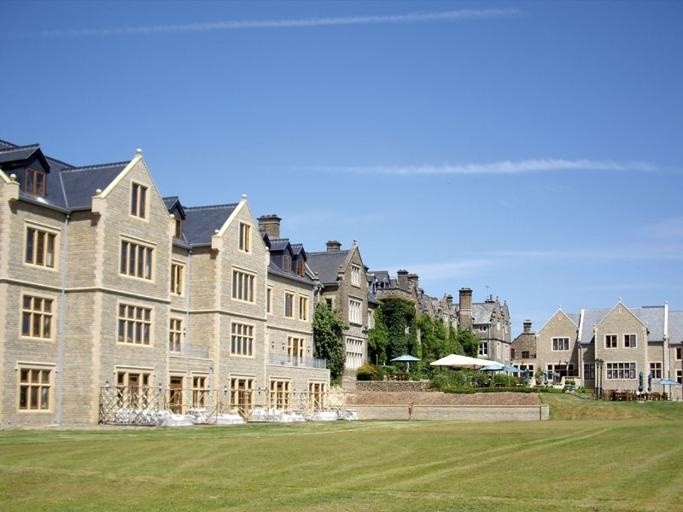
[504,364,525,375]
[427,353,504,373]
[389,353,422,372]
[638,369,644,403]
[480,364,506,375]
[543,371,549,388]
[648,370,653,401]
[657,377,680,401]
[552,370,558,387]
